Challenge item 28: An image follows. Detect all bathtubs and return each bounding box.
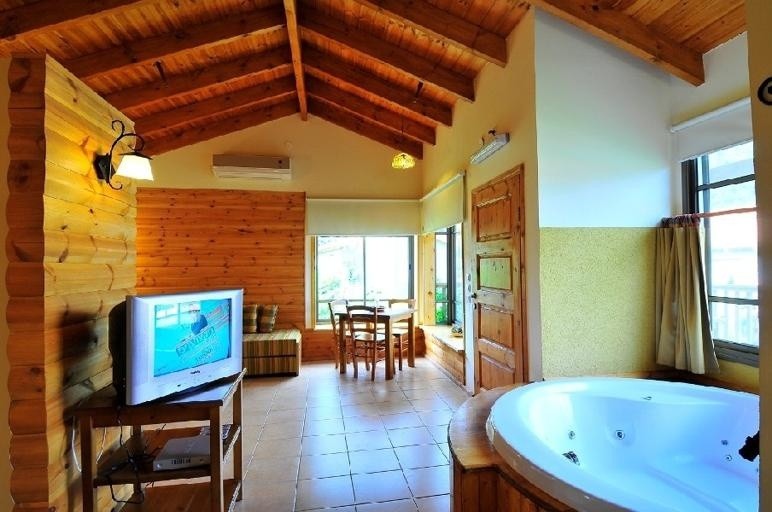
[485,377,760,512]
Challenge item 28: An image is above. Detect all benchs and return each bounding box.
[243,328,302,378]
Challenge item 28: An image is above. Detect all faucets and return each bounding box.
[738,430,760,461]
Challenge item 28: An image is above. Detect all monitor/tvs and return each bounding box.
[107,288,243,407]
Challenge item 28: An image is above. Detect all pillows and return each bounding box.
[244,304,279,333]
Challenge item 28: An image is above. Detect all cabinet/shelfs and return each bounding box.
[74,367,248,511]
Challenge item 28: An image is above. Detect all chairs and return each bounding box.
[329,298,415,381]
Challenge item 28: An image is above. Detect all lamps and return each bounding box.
[96,119,154,190]
[391,116,416,171]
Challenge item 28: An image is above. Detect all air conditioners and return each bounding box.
[211,152,292,181]
